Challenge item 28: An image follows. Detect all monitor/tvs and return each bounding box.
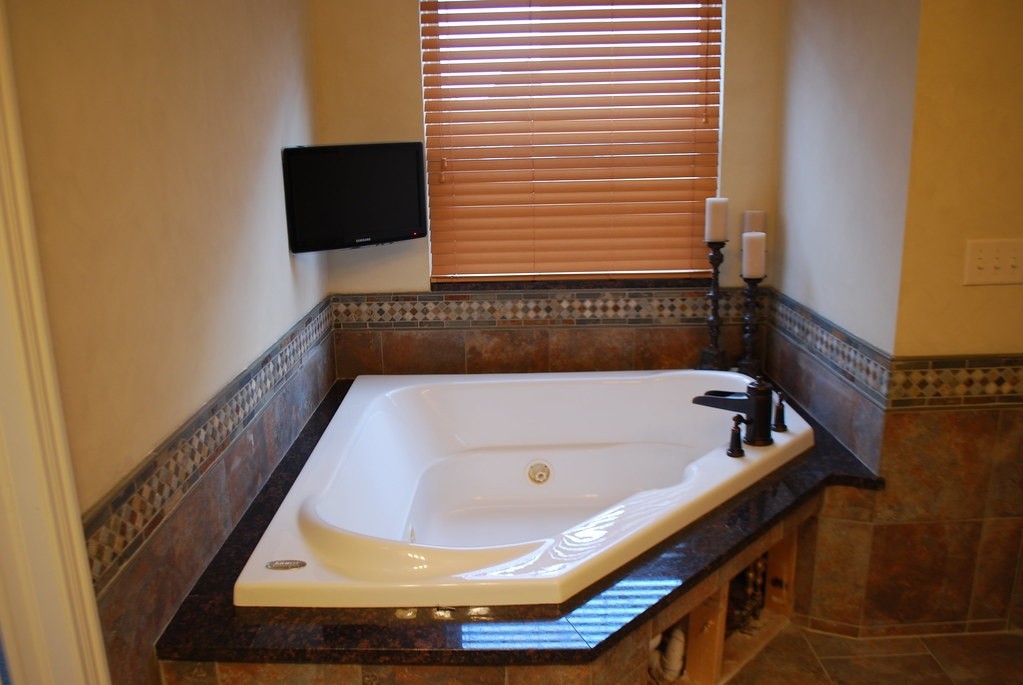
[282,141,428,252]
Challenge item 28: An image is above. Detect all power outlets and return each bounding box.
[963,238,1023,286]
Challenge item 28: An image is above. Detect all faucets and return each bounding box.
[692,375,774,447]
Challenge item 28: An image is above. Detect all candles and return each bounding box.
[742,232,767,278]
[742,210,766,232]
[704,197,729,241]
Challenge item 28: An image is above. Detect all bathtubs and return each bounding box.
[232,369,815,608]
